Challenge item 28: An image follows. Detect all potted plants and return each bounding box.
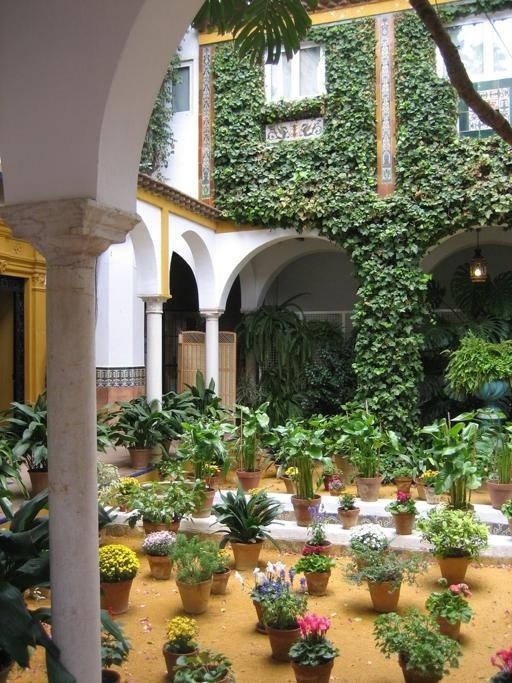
[0,365,512,682]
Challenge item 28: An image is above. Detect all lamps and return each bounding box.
[468,227,487,283]
